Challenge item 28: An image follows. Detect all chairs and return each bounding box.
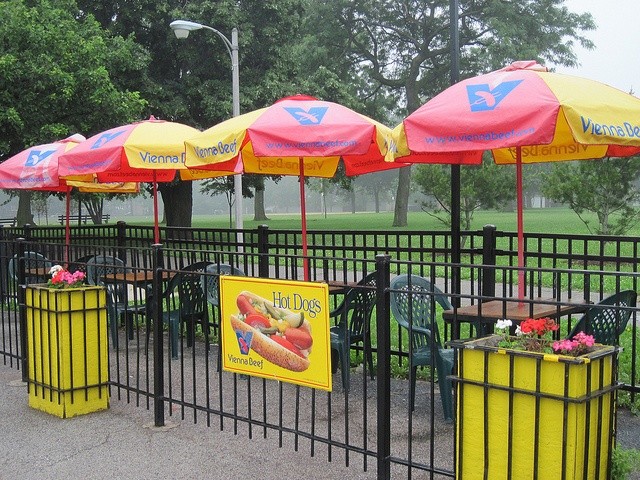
[201,265,245,307]
[87,255,125,349]
[147,262,215,360]
[64,255,93,282]
[9,252,51,281]
[330,271,377,389]
[567,289,638,339]
[391,275,455,425]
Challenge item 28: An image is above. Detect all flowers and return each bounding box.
[48,267,86,289]
[494,318,594,357]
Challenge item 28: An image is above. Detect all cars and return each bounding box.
[265,204,288,214]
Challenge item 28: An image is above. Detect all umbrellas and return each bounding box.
[184,94,412,283]
[0,133,139,267]
[384,60,640,307]
[57,116,239,244]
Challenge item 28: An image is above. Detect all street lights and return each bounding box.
[170,19,243,258]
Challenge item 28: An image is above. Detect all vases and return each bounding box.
[454,333,617,480]
[22,283,110,418]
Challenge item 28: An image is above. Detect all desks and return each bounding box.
[441,298,578,340]
[306,280,357,295]
[23,265,59,279]
[100,267,176,334]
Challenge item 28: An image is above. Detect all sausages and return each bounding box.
[97,136,109,151]
[475,89,498,110]
[30,153,39,170]
[296,111,319,127]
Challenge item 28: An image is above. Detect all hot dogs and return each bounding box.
[230,289,314,374]
